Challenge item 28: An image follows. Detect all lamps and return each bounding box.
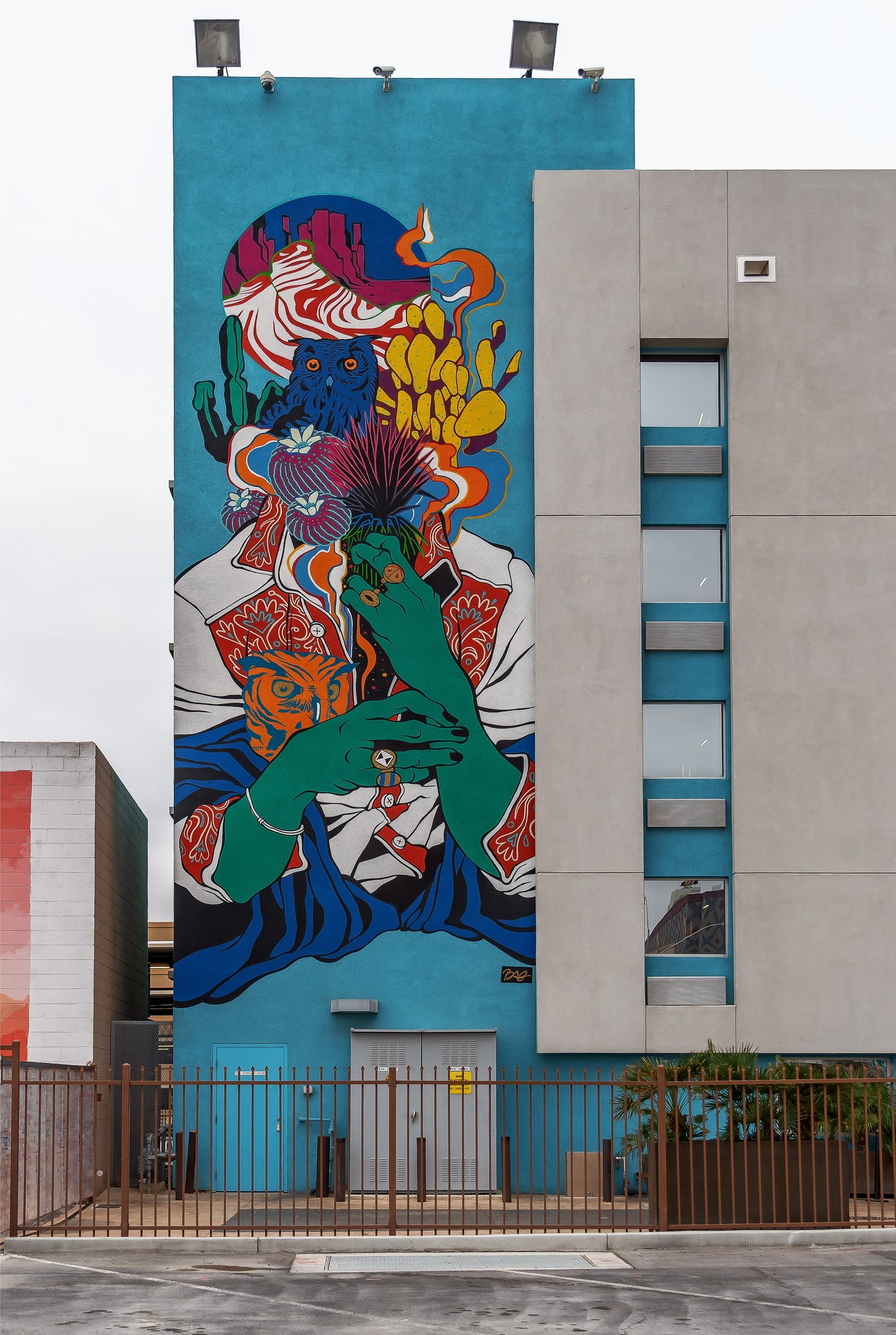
[510,20,559,78]
[193,19,241,75]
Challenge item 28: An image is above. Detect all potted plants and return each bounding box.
[610,1038,896,1227]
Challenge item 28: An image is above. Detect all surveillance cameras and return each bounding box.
[372,66,395,77]
[578,66,605,79]
[259,71,276,93]
